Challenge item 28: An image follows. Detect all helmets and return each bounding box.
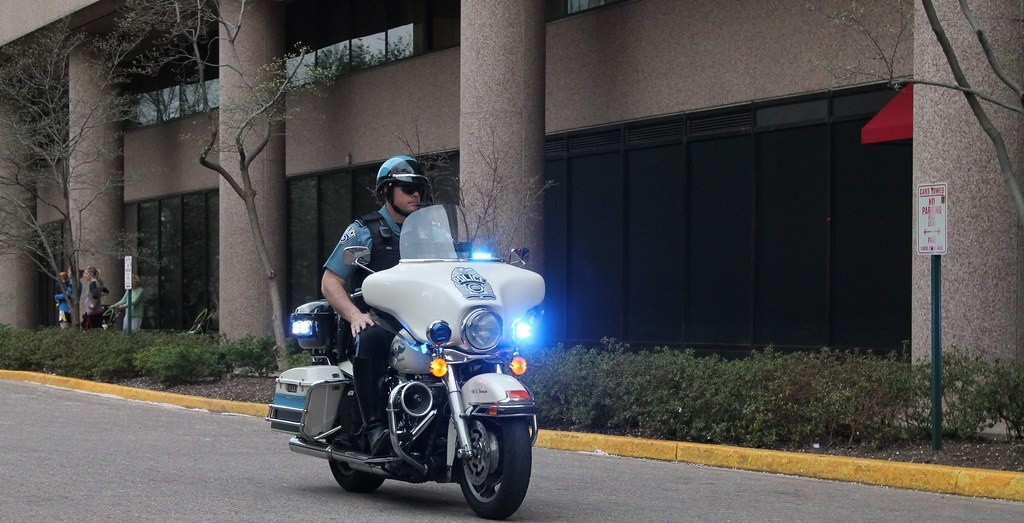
[376,156,428,193]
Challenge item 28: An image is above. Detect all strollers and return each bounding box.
[185,306,217,336]
[81,305,124,330]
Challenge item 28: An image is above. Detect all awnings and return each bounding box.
[862,81,913,143]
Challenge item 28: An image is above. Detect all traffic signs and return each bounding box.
[917,182,947,256]
[125,256,132,289]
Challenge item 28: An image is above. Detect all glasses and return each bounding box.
[392,183,425,195]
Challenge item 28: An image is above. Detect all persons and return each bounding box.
[54,265,84,330]
[320,156,447,458]
[110,274,145,331]
[82,265,109,329]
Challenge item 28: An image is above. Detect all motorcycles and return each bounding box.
[266,205,546,520]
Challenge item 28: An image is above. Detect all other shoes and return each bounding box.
[368,423,390,455]
